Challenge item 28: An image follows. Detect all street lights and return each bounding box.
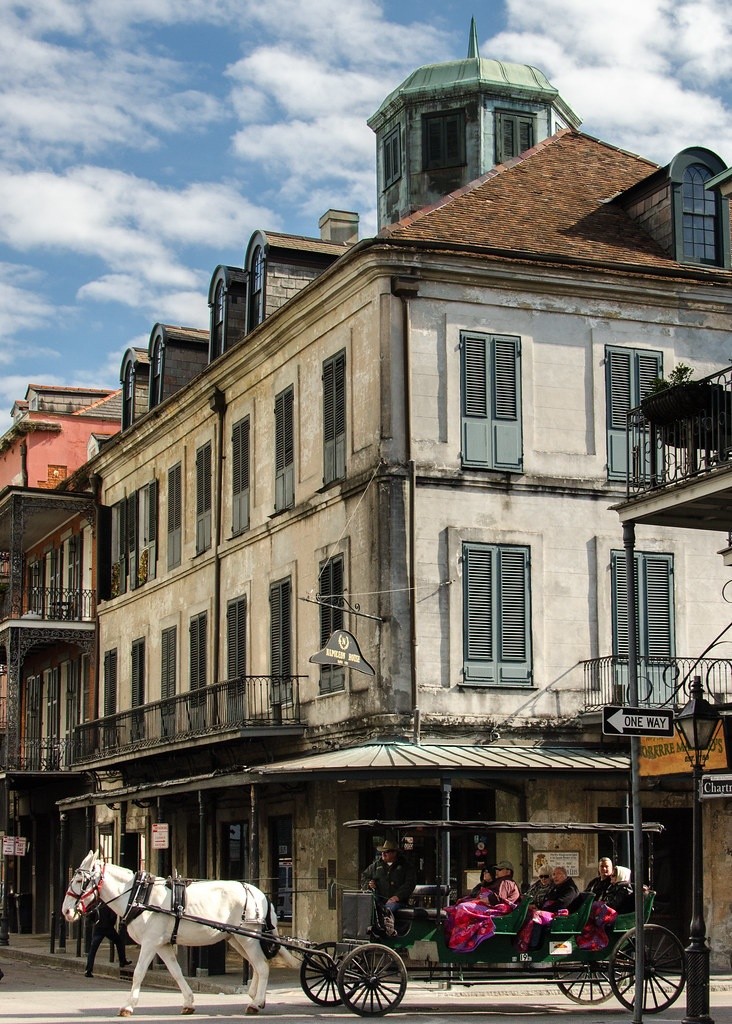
[672,675,723,1024]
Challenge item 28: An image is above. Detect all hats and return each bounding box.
[494,861,513,871]
[614,865,631,883]
[377,839,402,851]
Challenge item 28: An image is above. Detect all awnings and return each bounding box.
[54,767,313,812]
[0,770,90,786]
[243,739,634,774]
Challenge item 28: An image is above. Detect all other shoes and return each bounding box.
[514,941,528,953]
[85,970,93,977]
[119,960,132,967]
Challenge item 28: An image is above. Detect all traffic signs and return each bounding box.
[601,705,675,739]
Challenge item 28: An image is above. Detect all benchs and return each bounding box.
[413,884,451,909]
[492,895,533,936]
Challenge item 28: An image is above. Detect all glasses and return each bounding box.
[494,867,504,871]
[539,875,550,879]
[484,872,489,874]
[382,850,394,853]
[610,873,617,877]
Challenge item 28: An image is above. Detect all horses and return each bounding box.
[61,849,303,1017]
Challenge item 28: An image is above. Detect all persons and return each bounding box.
[511,866,581,952]
[474,841,488,860]
[84,902,132,977]
[575,856,650,952]
[360,841,417,937]
[443,860,521,953]
[8,886,23,933]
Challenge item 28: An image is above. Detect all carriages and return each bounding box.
[60,819,687,1019]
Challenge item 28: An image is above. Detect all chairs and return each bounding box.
[613,889,658,933]
[551,890,596,935]
